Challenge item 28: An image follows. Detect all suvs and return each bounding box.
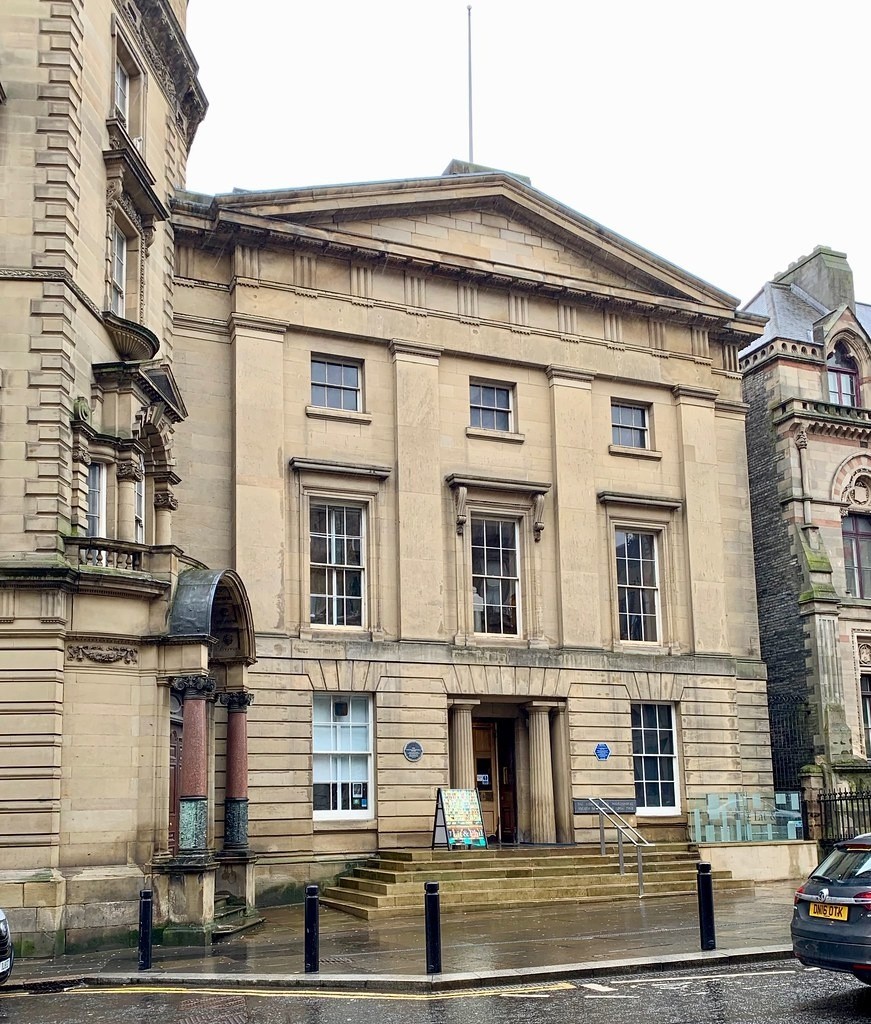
[790,833,871,987]
